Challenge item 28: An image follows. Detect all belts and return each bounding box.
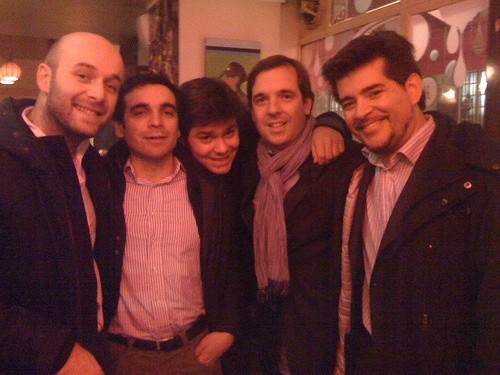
[100,317,208,351]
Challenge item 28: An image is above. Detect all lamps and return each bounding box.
[0,62,22,85]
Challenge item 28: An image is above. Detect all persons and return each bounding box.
[247,53,365,375]
[320,29,500,375]
[177,75,353,375]
[97,70,243,375]
[0,31,125,375]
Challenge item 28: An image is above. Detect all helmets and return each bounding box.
[226,62,246,82]
